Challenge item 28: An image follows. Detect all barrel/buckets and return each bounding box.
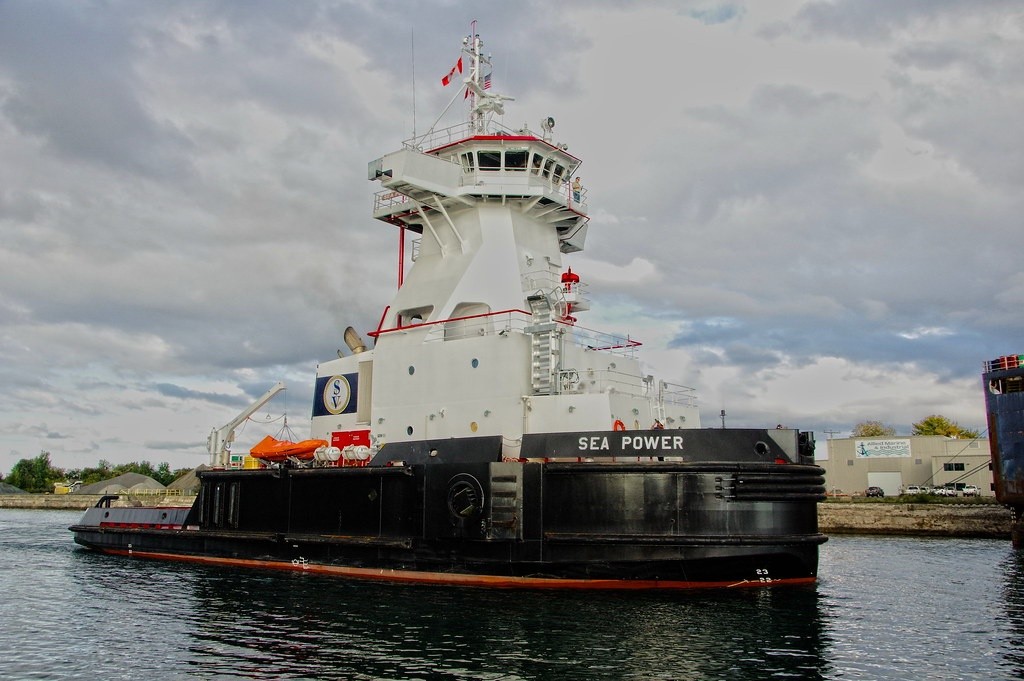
[985,354,1024,373]
[231,455,258,469]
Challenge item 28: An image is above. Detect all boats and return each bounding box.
[65,18,830,596]
[981,355,1024,550]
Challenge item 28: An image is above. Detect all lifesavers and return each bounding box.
[614,420,625,431]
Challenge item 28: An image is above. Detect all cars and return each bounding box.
[850,490,865,498]
[865,486,884,498]
[919,486,958,497]
[825,488,850,496]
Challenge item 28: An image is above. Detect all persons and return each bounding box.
[650,418,667,464]
[572,176,584,204]
[463,72,493,101]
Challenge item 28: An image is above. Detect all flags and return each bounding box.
[441,56,463,87]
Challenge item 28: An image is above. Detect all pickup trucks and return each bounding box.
[962,484,981,497]
[905,486,920,495]
